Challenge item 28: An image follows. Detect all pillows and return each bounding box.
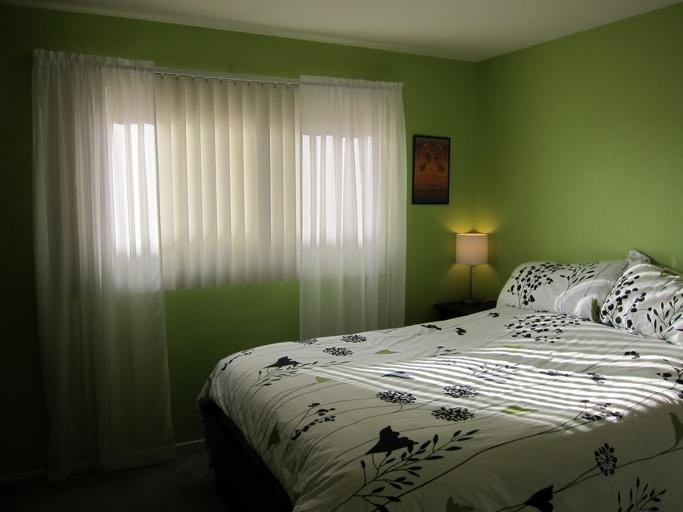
[495,259,629,323]
[599,251,682,348]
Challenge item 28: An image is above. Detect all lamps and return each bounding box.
[455,231,489,303]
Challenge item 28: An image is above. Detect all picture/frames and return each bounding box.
[411,135,450,205]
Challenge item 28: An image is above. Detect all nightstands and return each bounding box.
[432,298,496,321]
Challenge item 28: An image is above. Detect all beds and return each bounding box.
[194,247,681,512]
